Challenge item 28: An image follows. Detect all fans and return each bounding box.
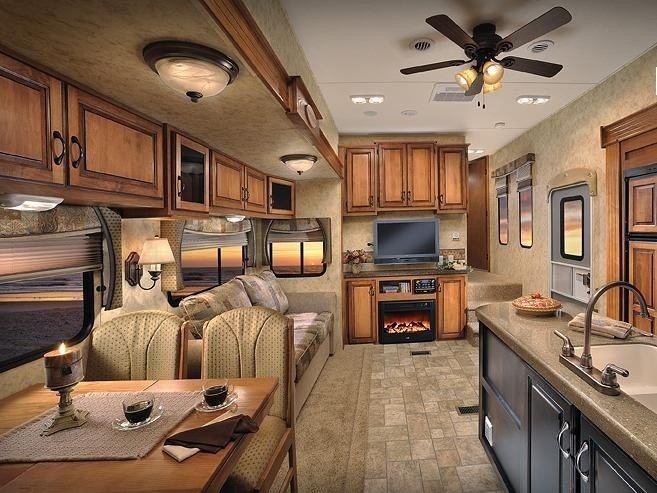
[399,4,573,98]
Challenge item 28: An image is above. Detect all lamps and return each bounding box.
[514,95,552,108]
[455,67,478,92]
[125,234,175,292]
[348,92,386,108]
[278,152,317,177]
[481,57,504,94]
[140,38,240,104]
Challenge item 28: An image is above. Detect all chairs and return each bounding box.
[198,305,299,493]
[84,309,191,381]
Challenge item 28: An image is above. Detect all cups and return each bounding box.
[202,378,229,409]
[122,392,155,423]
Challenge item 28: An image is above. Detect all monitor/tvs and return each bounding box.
[373,218,440,263]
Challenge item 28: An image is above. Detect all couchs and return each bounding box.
[179,270,338,424]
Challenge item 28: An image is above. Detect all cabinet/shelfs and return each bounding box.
[377,274,436,302]
[436,274,468,341]
[338,144,378,217]
[439,141,471,214]
[0,50,166,213]
[473,307,525,492]
[171,124,210,219]
[210,148,267,219]
[342,278,379,346]
[525,362,657,492]
[267,174,298,219]
[374,140,440,211]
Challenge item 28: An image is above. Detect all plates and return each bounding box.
[195,391,238,412]
[111,405,164,430]
[512,299,562,316]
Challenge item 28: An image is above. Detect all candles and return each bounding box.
[41,342,85,390]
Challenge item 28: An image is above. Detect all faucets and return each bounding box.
[580,282,649,370]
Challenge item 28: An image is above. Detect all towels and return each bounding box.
[164,413,260,454]
[568,312,633,339]
[161,410,241,463]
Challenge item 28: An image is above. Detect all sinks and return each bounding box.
[571,343,657,395]
